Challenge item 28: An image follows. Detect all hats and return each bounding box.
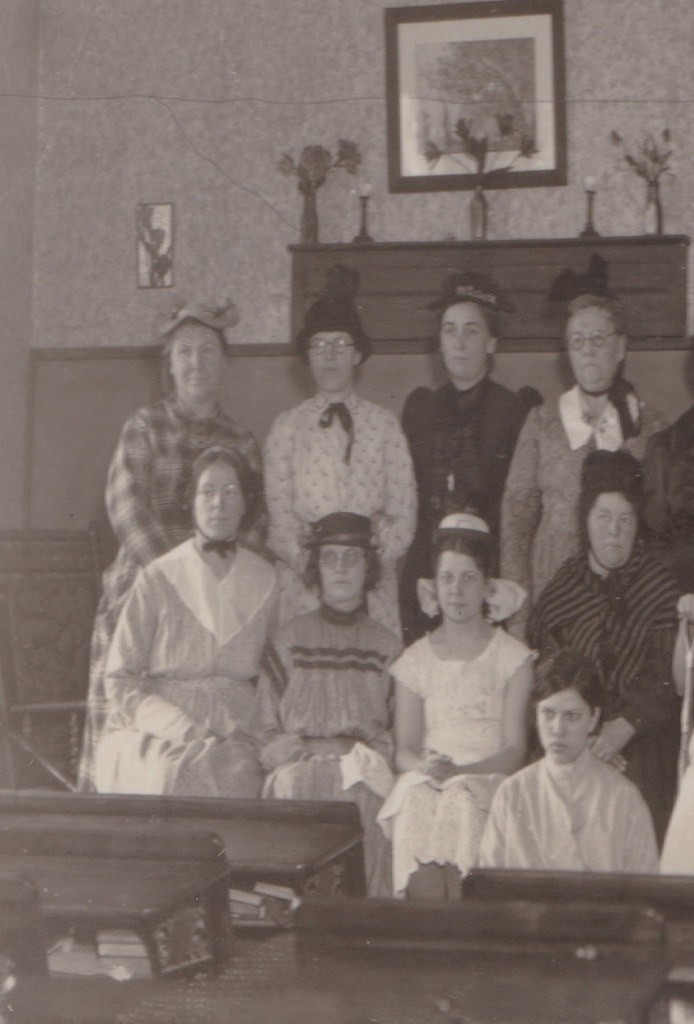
[294,296,366,349]
[300,511,376,551]
[578,449,643,501]
[424,273,513,315]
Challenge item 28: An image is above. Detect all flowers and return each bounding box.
[606,126,677,182]
[279,139,363,194]
[423,109,541,187]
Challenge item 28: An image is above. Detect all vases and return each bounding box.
[351,196,374,243]
[643,182,663,233]
[300,193,318,244]
[470,187,487,240]
[578,190,598,237]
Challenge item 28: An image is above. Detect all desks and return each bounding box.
[458,866,694,1024]
[0,822,238,979]
[0,789,366,964]
[293,898,676,1024]
[0,969,647,1024]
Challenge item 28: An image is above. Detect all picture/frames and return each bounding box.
[134,203,173,288]
[384,0,567,194]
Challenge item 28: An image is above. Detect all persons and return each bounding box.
[500,284,670,643]
[402,274,544,646]
[99,446,280,797]
[376,513,539,902]
[257,512,406,899]
[77,301,265,792]
[520,335,694,876]
[481,648,658,874]
[264,296,418,645]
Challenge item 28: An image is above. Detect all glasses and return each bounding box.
[567,330,618,351]
[194,485,245,502]
[315,550,365,568]
[305,336,358,354]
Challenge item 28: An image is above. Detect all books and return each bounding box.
[95,908,207,958]
[229,879,300,919]
